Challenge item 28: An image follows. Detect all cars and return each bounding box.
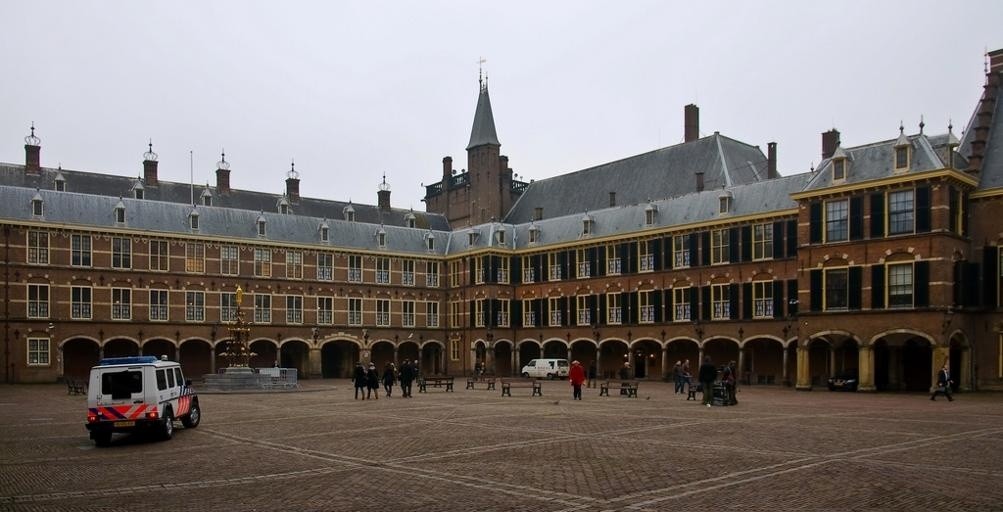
[825,366,860,391]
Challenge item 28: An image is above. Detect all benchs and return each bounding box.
[600,379,640,398]
[419,374,542,397]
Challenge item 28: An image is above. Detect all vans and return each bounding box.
[82,354,203,448]
[520,358,570,381]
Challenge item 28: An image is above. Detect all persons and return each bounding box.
[673,354,738,407]
[472,358,481,381]
[270,360,280,385]
[588,360,598,388]
[617,361,630,396]
[930,363,954,401]
[480,360,486,381]
[351,357,414,399]
[569,360,586,400]
[412,359,419,377]
